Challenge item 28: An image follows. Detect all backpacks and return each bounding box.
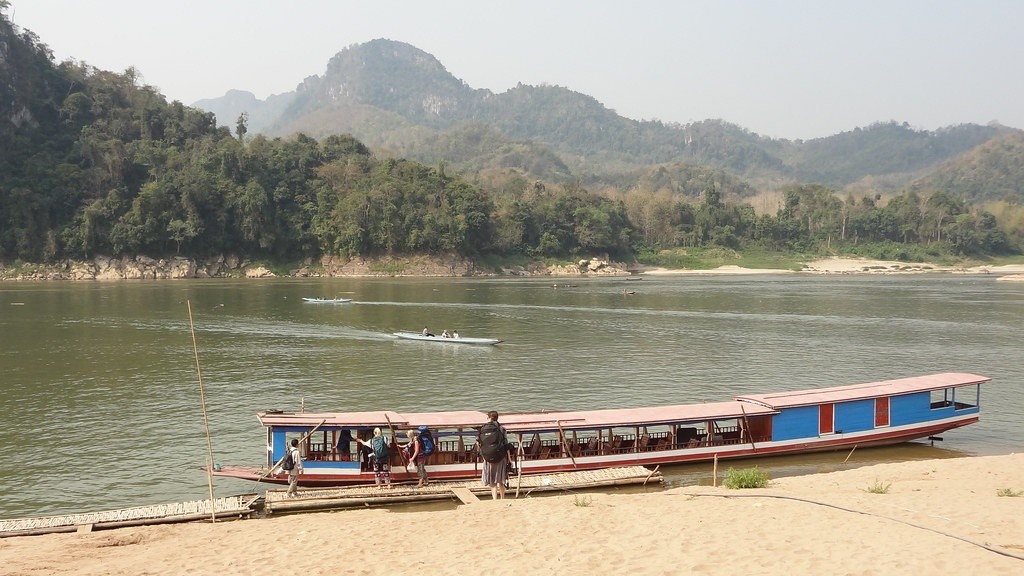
[282,448,296,470]
[480,421,512,463]
[412,426,435,458]
[373,436,389,464]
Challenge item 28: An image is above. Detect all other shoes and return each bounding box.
[284,493,301,499]
[415,485,422,488]
[422,483,429,486]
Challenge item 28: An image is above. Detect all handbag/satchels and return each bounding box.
[407,462,416,471]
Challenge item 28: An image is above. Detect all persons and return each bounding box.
[355,427,393,489]
[422,326,435,337]
[336,430,355,461]
[442,329,459,339]
[284,439,303,497]
[475,411,513,500]
[396,429,429,487]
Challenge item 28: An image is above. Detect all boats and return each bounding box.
[199,372,992,481]
[393,332,504,345]
[302,298,352,303]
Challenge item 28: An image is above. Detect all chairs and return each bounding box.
[460,429,722,464]
